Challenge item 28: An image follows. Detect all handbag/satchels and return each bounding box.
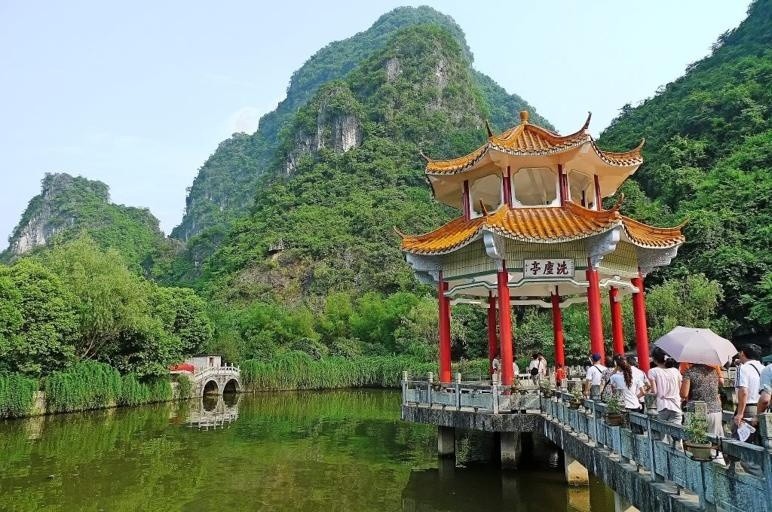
[532,368,538,375]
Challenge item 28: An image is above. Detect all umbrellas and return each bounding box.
[655,326,737,367]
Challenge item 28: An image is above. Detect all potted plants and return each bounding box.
[543,382,552,398]
[539,378,547,391]
[568,386,583,409]
[432,375,440,390]
[442,383,448,393]
[685,403,713,461]
[601,391,624,426]
[512,378,520,393]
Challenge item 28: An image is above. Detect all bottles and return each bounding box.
[740,420,757,434]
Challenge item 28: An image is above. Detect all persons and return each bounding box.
[489,351,565,388]
[584,344,772,446]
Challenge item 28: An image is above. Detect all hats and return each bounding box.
[593,353,601,360]
